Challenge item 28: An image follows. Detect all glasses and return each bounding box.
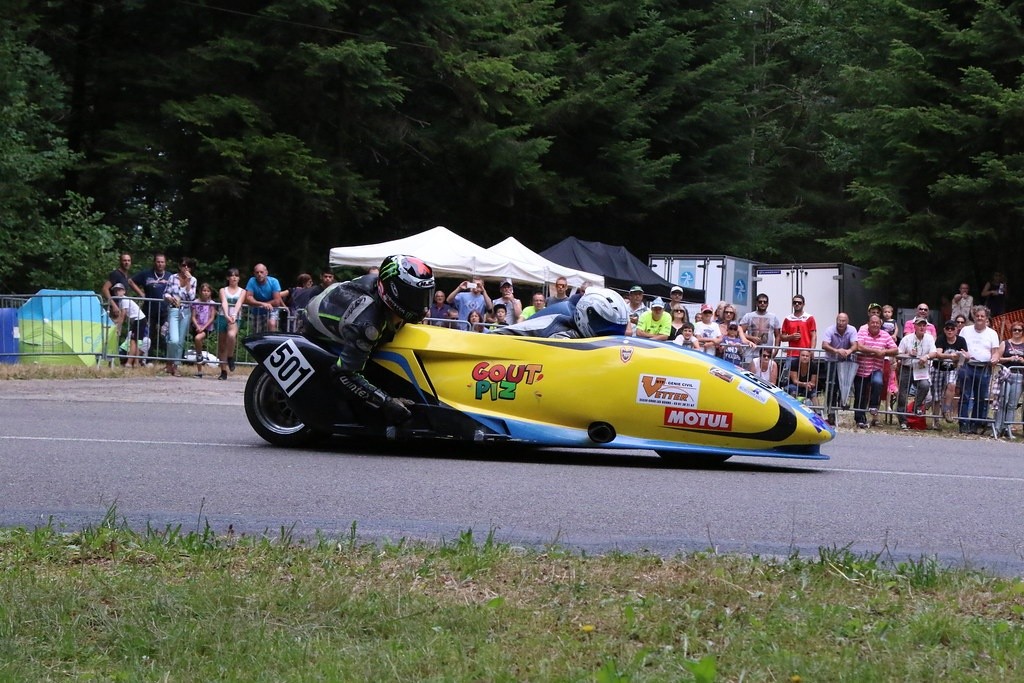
[763,354,771,358]
[631,291,642,295]
[1012,328,1022,332]
[702,310,712,314]
[918,308,928,313]
[725,300,803,331]
[182,264,192,268]
[556,283,566,288]
[945,327,956,331]
[673,309,684,313]
[956,321,964,324]
[672,291,682,296]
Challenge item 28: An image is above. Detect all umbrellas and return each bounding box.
[836,354,859,412]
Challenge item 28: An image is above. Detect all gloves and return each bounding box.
[382,397,416,421]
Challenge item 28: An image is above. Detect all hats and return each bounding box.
[944,320,957,328]
[629,286,644,295]
[651,299,665,309]
[111,283,125,290]
[868,303,883,313]
[670,286,683,296]
[701,304,713,313]
[499,277,513,288]
[912,318,927,325]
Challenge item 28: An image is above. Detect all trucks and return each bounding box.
[751,263,876,363]
[645,254,762,323]
[897,308,943,339]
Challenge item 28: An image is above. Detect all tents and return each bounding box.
[329,226,709,304]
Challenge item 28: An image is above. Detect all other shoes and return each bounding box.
[125,356,235,380]
[809,400,1024,439]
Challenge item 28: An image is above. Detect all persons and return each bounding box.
[302,254,435,424]
[823,303,937,432]
[980,270,1007,316]
[952,283,973,326]
[487,286,629,337]
[930,306,1024,440]
[102,254,214,379]
[280,266,379,332]
[245,263,280,332]
[217,268,246,381]
[624,285,821,418]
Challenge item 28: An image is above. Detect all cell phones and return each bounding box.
[467,283,477,288]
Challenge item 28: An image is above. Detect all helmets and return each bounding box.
[376,254,435,324]
[574,287,629,340]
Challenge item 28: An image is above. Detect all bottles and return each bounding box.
[797,326,801,340]
[757,333,764,345]
[914,342,918,358]
